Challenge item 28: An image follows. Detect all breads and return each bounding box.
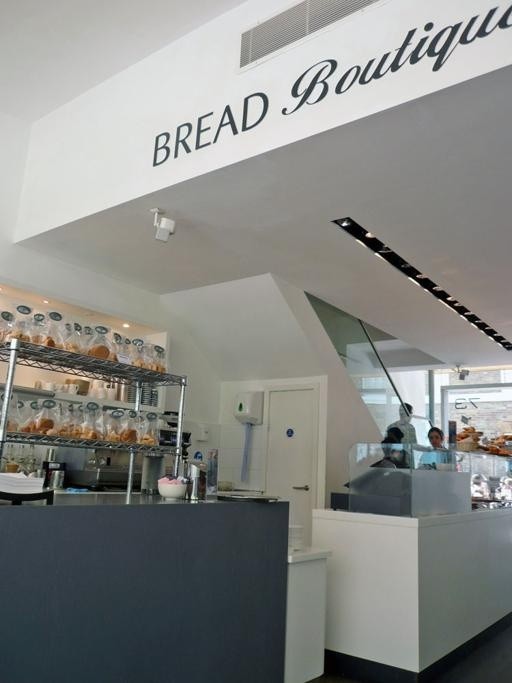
[0,328,166,447]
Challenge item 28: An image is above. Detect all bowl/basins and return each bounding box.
[157,482,188,501]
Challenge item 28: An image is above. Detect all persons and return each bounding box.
[387,403,432,468]
[388,426,411,468]
[374,437,402,468]
[418,426,457,471]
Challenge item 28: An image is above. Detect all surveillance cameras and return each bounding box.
[461,370,469,375]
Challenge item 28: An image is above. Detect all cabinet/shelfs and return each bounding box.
[284,546,331,683]
[0,339,189,493]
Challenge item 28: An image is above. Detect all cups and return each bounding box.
[49,470,65,489]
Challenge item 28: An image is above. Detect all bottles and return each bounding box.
[2,443,37,475]
